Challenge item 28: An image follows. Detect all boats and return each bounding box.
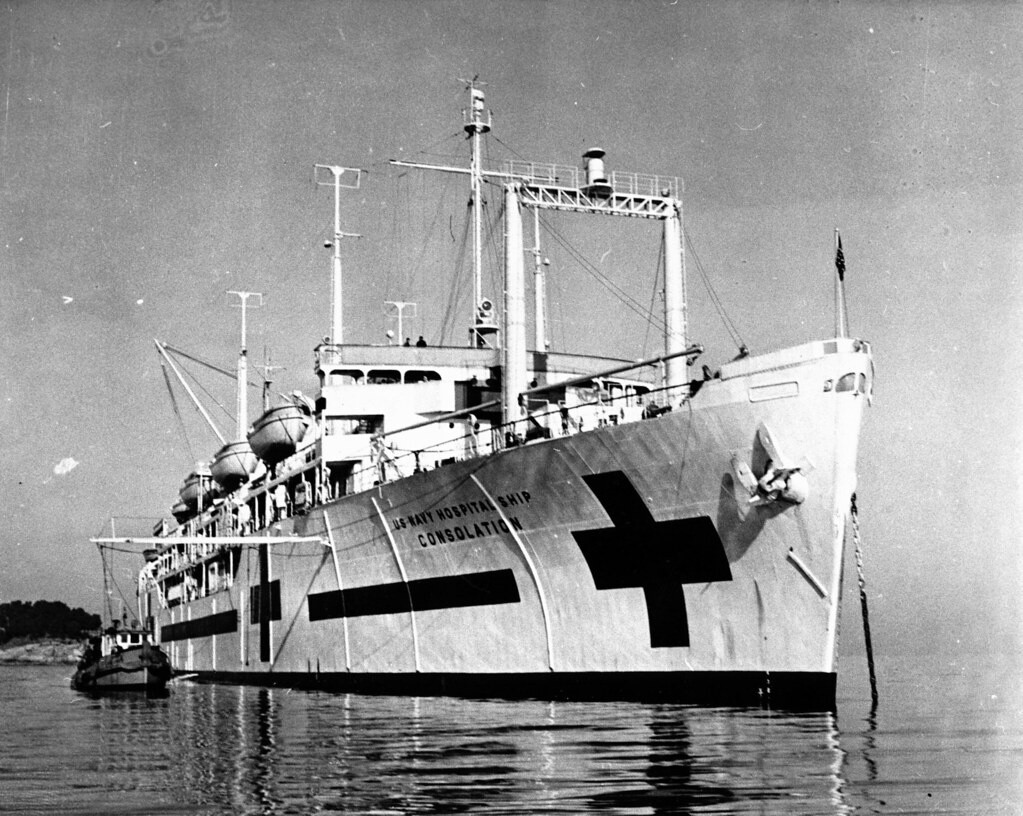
[69,588,173,700]
[83,70,887,719]
